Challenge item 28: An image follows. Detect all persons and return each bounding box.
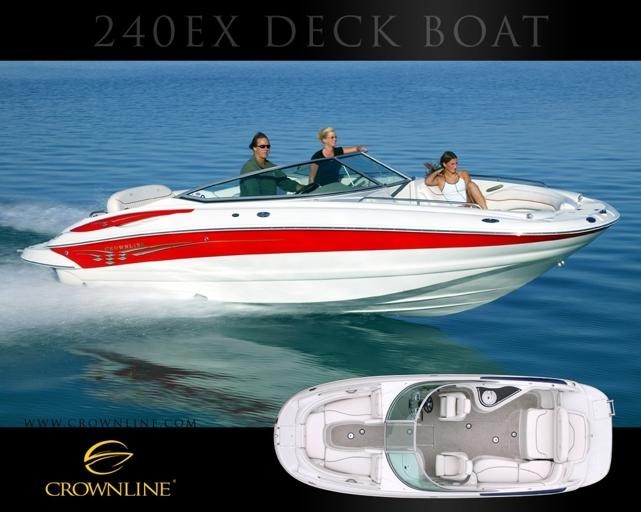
[424,151,487,209]
[310,128,368,184]
[238,132,303,195]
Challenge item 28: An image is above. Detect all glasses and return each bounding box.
[257,145,271,149]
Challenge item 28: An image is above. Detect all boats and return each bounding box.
[66,316,514,426]
[19,150,622,319]
[274,371,616,500]
